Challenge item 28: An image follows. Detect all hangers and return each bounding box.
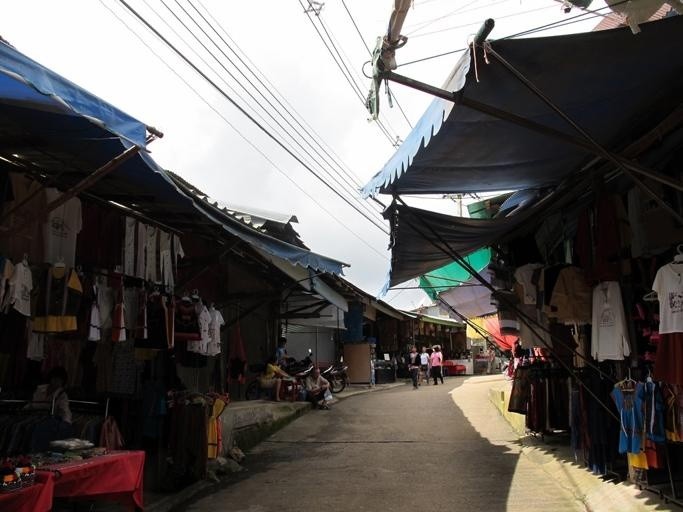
[598,247,683,393]
[164,386,229,409]
[0,397,109,428]
[0,164,216,313]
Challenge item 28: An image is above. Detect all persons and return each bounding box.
[305,367,331,410]
[419,346,430,386]
[428,344,444,384]
[259,356,296,402]
[20,367,72,427]
[485,346,496,373]
[274,336,289,370]
[408,346,421,389]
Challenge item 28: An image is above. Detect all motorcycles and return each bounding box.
[243,347,349,399]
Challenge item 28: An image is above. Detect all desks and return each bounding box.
[0,448,146,512]
